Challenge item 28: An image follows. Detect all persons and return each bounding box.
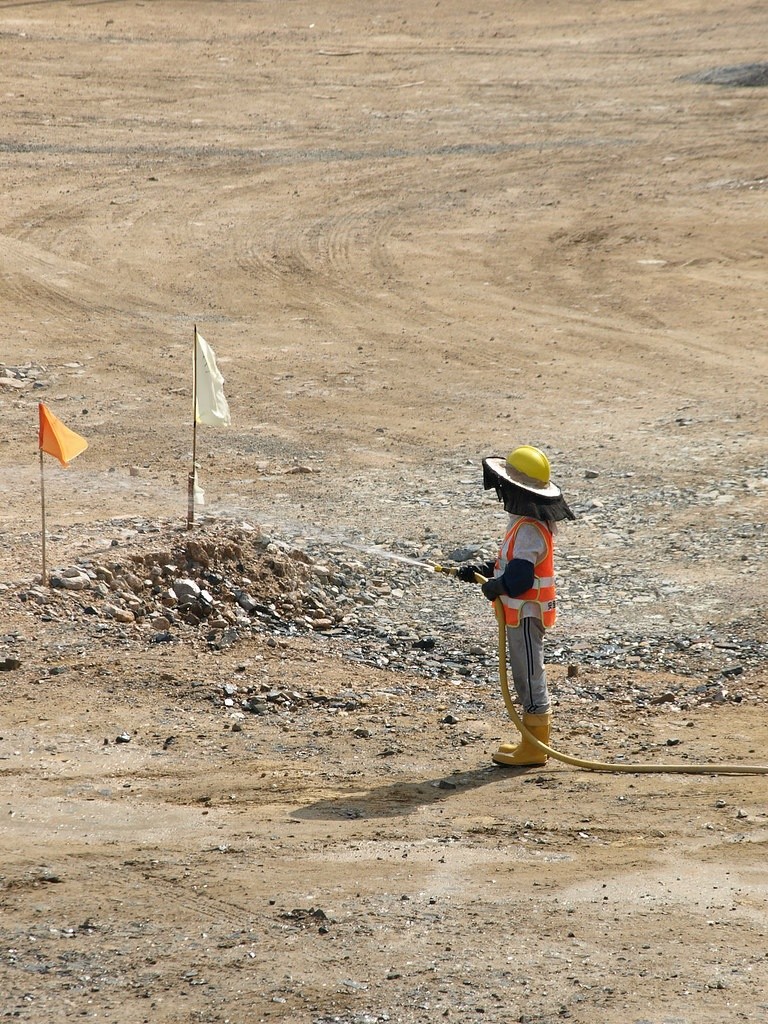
[458,445,576,766]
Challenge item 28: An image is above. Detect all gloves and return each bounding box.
[482,577,506,601]
[456,565,485,584]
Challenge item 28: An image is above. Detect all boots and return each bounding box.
[491,711,552,765]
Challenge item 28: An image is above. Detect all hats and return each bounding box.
[482,446,576,523]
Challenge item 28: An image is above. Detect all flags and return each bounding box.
[193,332,231,426]
[38,404,89,464]
